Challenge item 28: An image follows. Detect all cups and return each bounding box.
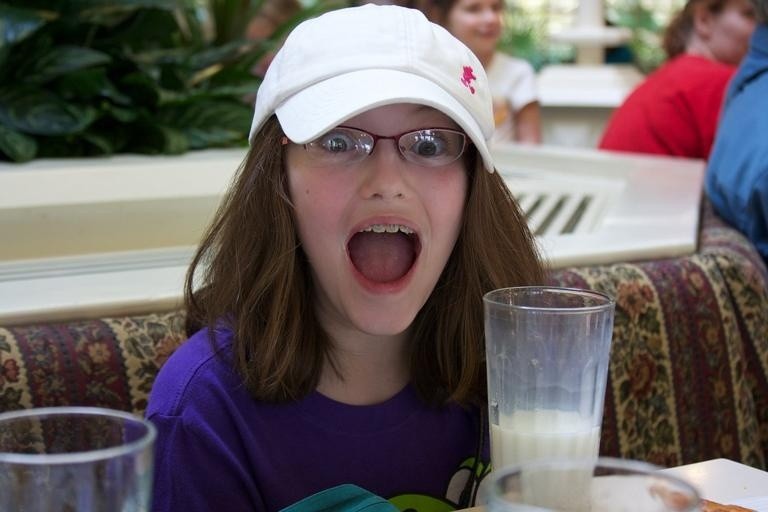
[1,406,158,512]
[479,458,701,512]
[480,285,619,512]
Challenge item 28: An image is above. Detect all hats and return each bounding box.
[249,3,496,174]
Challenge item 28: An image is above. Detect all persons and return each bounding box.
[704,0,768,263]
[599,0,757,159]
[439,0,540,143]
[145,4,554,512]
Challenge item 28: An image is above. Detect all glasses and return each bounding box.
[304,126,471,168]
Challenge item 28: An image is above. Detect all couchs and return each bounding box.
[2,148,768,511]
